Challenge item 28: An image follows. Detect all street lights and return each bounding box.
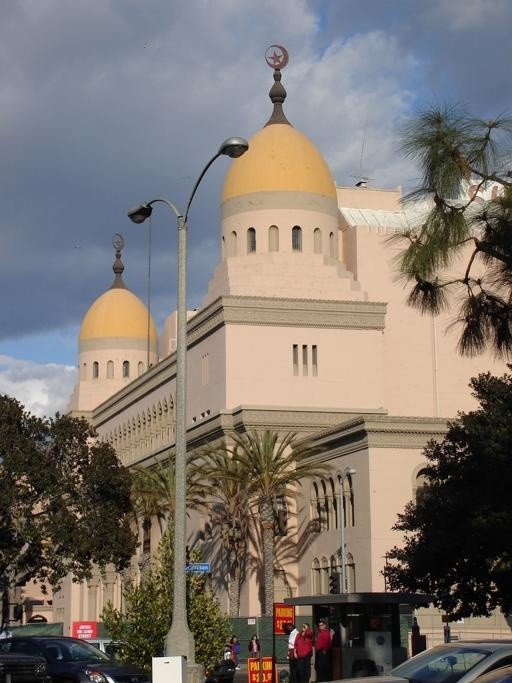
[127,134,251,683]
[340,464,355,595]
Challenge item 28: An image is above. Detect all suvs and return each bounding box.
[0,636,151,682]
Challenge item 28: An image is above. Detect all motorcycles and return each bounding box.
[202,647,237,683]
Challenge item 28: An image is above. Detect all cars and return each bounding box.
[319,638,512,682]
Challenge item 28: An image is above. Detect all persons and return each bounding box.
[223,620,333,682]
